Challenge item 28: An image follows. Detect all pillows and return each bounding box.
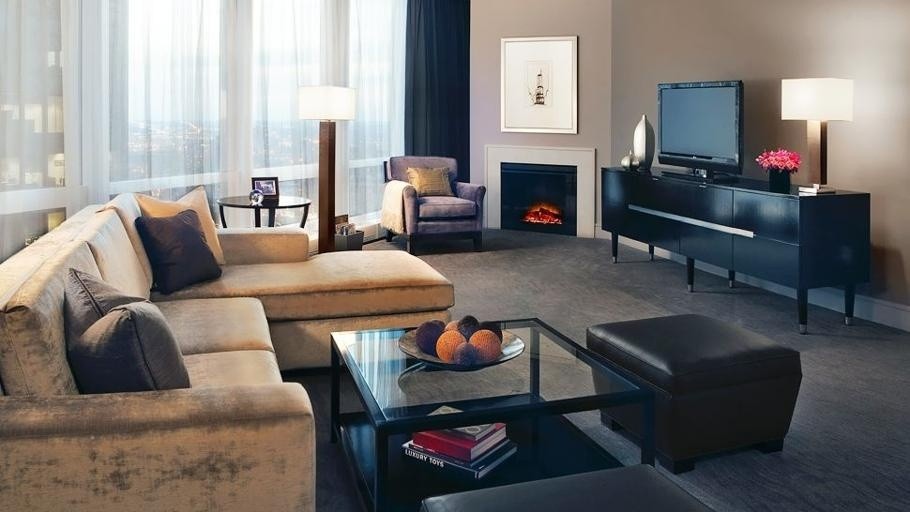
[133,186,225,265]
[134,209,221,295]
[407,165,453,196]
[64,267,190,392]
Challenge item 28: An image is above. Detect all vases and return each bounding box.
[768,170,790,190]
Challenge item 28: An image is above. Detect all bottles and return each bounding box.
[620,113,655,173]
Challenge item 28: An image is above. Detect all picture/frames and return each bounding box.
[501,35,578,135]
[252,176,279,199]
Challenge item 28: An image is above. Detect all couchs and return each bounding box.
[0,192,455,512]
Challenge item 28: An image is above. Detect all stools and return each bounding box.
[419,463,714,512]
[586,313,802,474]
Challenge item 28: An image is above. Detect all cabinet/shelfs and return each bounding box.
[601,166,870,333]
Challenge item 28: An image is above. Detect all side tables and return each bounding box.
[216,195,312,228]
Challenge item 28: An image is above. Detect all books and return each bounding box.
[400,406,517,480]
[336,222,356,235]
[799,186,836,194]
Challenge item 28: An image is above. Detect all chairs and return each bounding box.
[383,156,486,254]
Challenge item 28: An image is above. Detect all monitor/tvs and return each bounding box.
[659,81,744,183]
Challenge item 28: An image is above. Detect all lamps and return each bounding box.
[782,78,855,193]
[298,86,355,252]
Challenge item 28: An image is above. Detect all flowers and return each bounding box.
[755,148,802,174]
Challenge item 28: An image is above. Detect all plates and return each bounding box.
[396,327,527,369]
